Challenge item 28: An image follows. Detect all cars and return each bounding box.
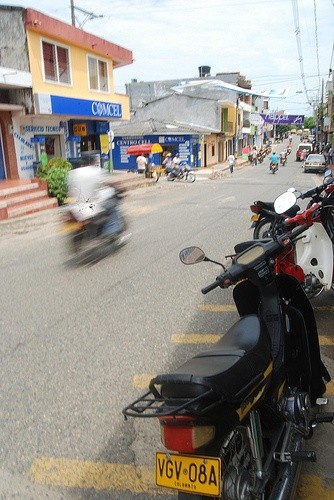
[303,154,327,173]
[291,129,316,161]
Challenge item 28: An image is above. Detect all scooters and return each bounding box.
[225,191,334,299]
[248,170,334,243]
[123,191,334,500]
[60,184,129,264]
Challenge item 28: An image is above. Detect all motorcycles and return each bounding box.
[270,145,291,174]
[165,162,196,183]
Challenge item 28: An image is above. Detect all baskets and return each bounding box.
[269,242,297,275]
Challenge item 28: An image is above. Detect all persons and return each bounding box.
[328,146,334,164]
[136,153,147,175]
[296,141,332,162]
[228,151,238,173]
[269,152,280,170]
[162,153,180,179]
[280,149,287,163]
[67,155,121,247]
[250,146,257,167]
